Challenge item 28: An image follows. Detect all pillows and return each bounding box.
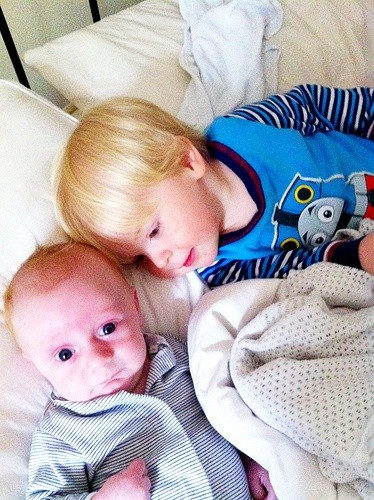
[1,81,100,313]
[25,0,374,137]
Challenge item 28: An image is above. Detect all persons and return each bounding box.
[55,84,374,291]
[3,242,277,500]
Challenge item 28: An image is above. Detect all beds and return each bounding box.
[1,0,374,499]
